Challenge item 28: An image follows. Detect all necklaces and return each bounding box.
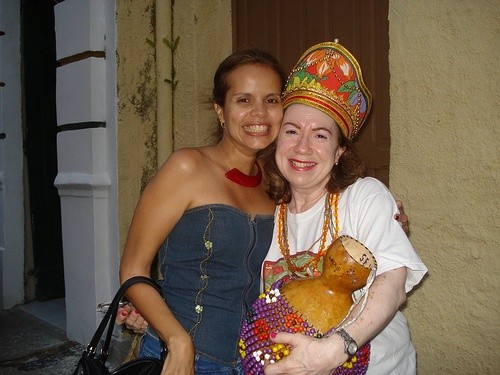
[277,191,340,278]
[224,160,263,188]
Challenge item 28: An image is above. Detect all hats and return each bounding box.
[281,38,373,141]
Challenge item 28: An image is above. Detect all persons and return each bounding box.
[116,41,429,375]
[119,47,412,375]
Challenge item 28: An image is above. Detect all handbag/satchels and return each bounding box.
[72,276,165,375]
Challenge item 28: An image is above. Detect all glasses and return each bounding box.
[96,300,128,314]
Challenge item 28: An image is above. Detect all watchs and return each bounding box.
[334,327,358,361]
